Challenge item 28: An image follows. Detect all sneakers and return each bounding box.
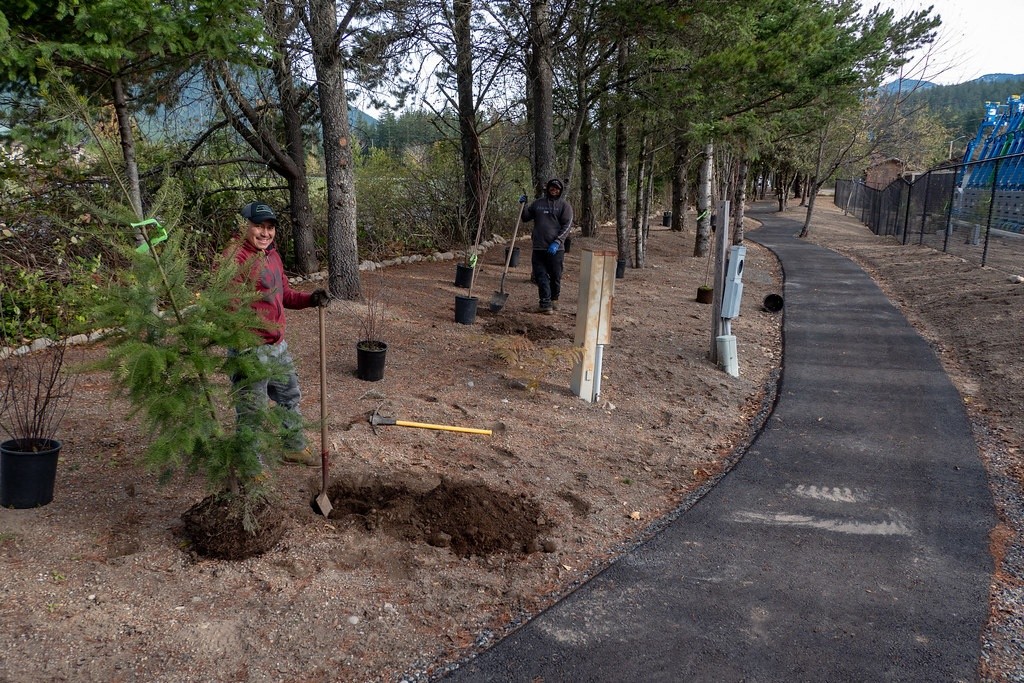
[278,446,323,468]
[526,303,553,314]
[554,301,562,311]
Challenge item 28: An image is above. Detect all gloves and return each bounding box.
[519,195,528,204]
[310,288,331,308]
[547,241,560,255]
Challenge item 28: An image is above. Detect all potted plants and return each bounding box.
[453,226,475,288]
[696,240,714,304]
[663,211,671,226]
[0,268,71,510]
[454,252,479,325]
[356,236,388,381]
[616,228,632,278]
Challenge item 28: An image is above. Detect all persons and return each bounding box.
[210,202,332,490]
[519,179,574,314]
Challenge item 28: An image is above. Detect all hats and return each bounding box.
[241,201,278,226]
[551,180,562,189]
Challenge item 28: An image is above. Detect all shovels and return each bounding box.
[491,200,525,314]
[315,300,332,518]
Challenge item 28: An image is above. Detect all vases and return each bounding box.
[564,237,571,253]
[764,292,783,313]
[504,247,519,266]
